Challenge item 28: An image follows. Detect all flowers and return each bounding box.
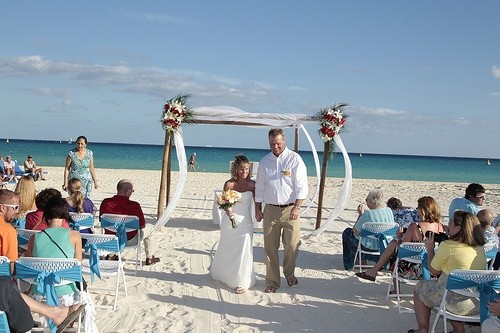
[217,190,243,227]
[317,104,348,141]
[159,96,195,132]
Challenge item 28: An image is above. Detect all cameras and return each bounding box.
[424,231,448,246]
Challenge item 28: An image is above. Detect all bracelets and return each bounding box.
[295,206,300,210]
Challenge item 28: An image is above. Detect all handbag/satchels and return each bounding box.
[75,276,87,291]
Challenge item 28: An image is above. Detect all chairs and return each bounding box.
[353,208,500,333]
[0,159,143,333]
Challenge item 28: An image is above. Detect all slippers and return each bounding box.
[389,291,401,298]
[56,303,87,333]
[355,272,375,281]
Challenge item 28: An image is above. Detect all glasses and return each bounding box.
[132,190,134,192]
[475,196,484,200]
[0,204,19,210]
[416,207,421,211]
[449,219,455,225]
[366,198,369,202]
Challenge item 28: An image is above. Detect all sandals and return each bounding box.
[265,286,276,293]
[286,276,298,286]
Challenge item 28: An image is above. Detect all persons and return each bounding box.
[27,197,82,328]
[15,176,36,229]
[26,188,72,230]
[0,155,18,189]
[0,189,21,274]
[449,183,500,271]
[255,128,308,292]
[209,156,263,293]
[98,180,160,265]
[63,136,97,197]
[355,196,447,300]
[62,178,95,257]
[342,192,419,270]
[481,297,500,333]
[407,210,486,333]
[188,153,197,172]
[0,275,87,333]
[24,155,47,181]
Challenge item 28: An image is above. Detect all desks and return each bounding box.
[211,190,268,228]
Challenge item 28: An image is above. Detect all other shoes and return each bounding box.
[146,256,160,265]
[106,254,116,260]
[408,329,417,333]
[237,287,245,294]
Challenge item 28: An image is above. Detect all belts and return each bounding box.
[270,203,294,208]
